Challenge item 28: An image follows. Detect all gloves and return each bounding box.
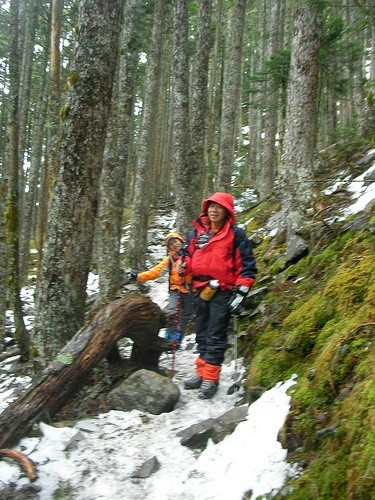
[129,274,137,280]
[225,285,249,314]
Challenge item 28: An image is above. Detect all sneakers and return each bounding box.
[185,375,202,389]
[198,382,217,399]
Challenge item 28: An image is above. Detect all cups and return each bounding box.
[199,280,220,301]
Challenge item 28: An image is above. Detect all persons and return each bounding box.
[124,232,192,354]
[172,192,258,400]
